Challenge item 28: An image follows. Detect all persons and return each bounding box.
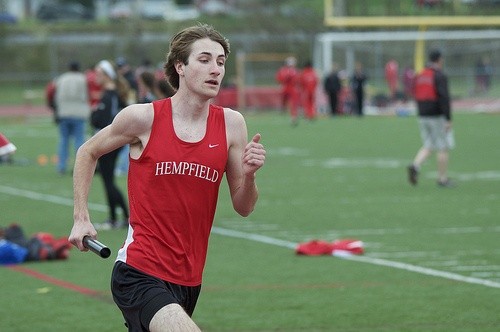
[46,25,266,332]
[406,48,456,188]
[277,52,493,127]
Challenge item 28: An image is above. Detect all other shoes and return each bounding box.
[94,218,129,231]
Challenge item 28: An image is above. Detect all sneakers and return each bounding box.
[408,164,418,186]
[437,179,455,189]
